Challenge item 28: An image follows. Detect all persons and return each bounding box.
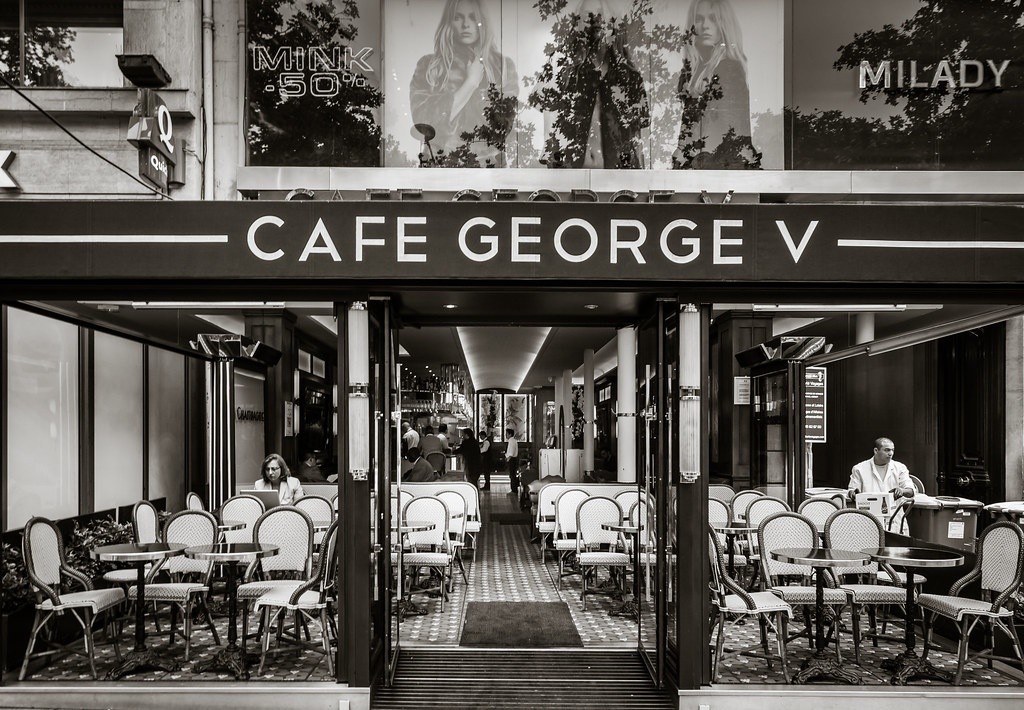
[479,431,494,491]
[534,0,651,169]
[296,420,326,464]
[410,0,519,168]
[453,428,481,509]
[299,450,331,483]
[253,454,304,506]
[595,448,617,480]
[402,447,437,482]
[848,438,918,536]
[504,429,519,496]
[672,0,764,170]
[400,421,452,476]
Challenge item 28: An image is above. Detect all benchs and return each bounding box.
[391,480,482,563]
[536,481,646,563]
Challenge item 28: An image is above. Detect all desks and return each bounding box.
[91,541,188,682]
[771,547,873,686]
[370,514,436,621]
[860,547,965,686]
[601,519,645,623]
[311,518,333,535]
[214,520,247,533]
[711,523,761,579]
[184,542,280,678]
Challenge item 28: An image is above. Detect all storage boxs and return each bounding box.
[904,491,984,554]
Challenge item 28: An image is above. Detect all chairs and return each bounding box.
[671,483,848,591]
[917,522,1024,688]
[757,512,843,685]
[610,489,658,552]
[824,509,928,664]
[706,524,793,686]
[106,492,338,679]
[573,496,629,613]
[626,502,657,611]
[369,487,469,620]
[551,489,602,590]
[17,516,129,682]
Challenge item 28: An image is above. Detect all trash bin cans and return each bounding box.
[903,496,985,553]
[805,487,856,508]
[983,502,1024,534]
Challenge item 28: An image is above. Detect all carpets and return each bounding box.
[459,600,584,649]
